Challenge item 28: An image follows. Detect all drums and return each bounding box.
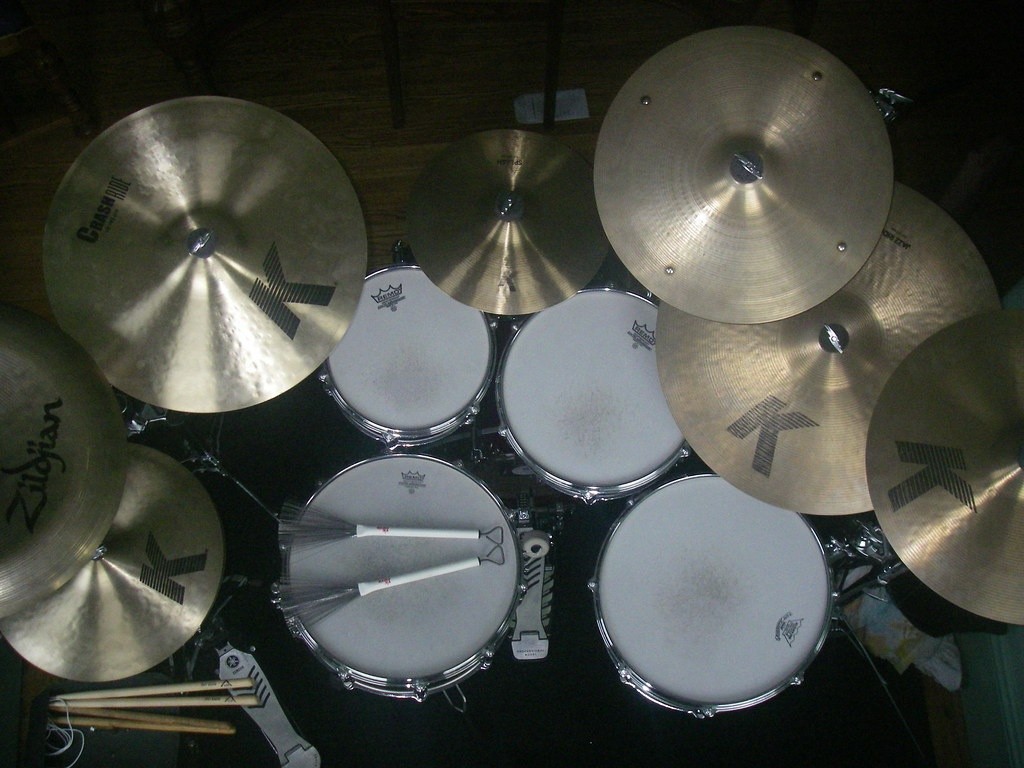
[493,286,690,503]
[592,472,840,718]
[283,454,523,701]
[322,266,502,442]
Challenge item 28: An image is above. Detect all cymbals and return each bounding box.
[656,177,1007,516]
[864,309,1024,628]
[42,94,370,416]
[592,24,891,325]
[0,304,129,620]
[1,441,227,682]
[404,127,615,316]
